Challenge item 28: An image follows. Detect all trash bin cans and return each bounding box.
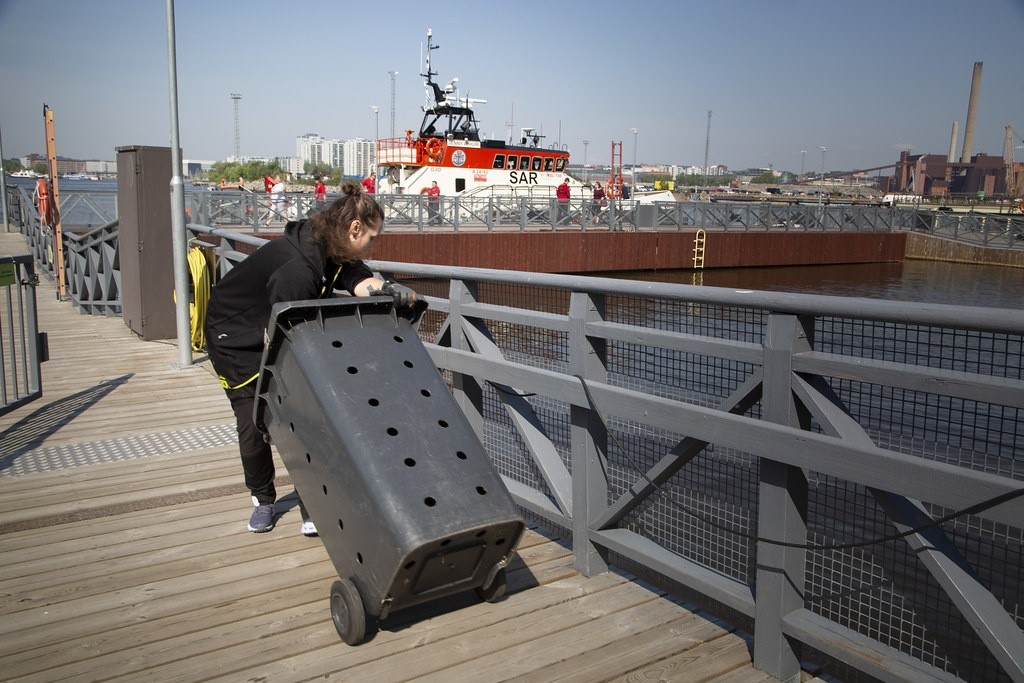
[254,296,525,646]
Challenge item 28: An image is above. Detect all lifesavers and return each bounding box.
[605,178,622,199]
[419,187,432,195]
[425,138,444,158]
[35,178,51,226]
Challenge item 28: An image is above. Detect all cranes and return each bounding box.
[1005,124,1024,204]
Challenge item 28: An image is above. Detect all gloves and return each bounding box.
[380,275,417,308]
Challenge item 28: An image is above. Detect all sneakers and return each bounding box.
[298,516,319,535]
[245,502,276,534]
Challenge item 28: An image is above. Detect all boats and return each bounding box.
[375,28,685,222]
[10,168,45,178]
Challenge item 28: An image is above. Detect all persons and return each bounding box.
[360,172,380,194]
[427,181,443,225]
[593,182,605,223]
[556,178,571,224]
[314,176,326,203]
[265,175,289,226]
[203,181,416,535]
